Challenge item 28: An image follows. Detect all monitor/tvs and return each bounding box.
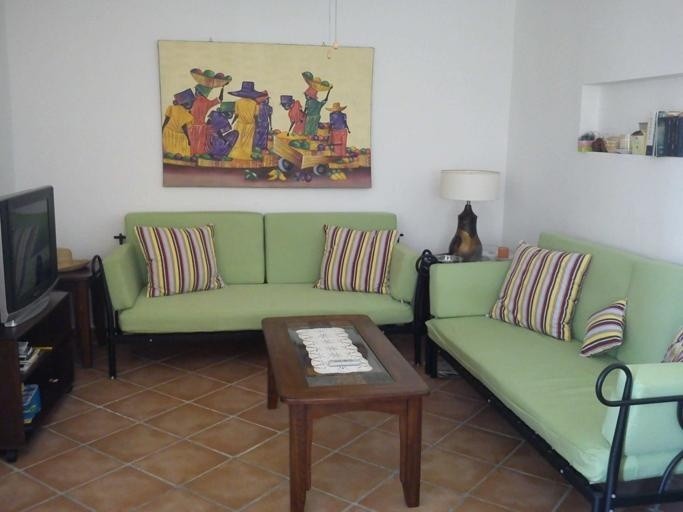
[0,184,61,326]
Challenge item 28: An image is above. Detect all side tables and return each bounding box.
[413,244,515,365]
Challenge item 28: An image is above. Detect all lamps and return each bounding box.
[440,169,501,261]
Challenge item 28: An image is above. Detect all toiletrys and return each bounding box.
[607,136,618,154]
[638,122,647,147]
[619,133,631,154]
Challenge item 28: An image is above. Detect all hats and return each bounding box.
[195,83,213,98]
[57,246,89,274]
[305,87,318,100]
[280,95,293,104]
[174,88,196,105]
[228,81,265,99]
[220,101,236,113]
[325,102,347,112]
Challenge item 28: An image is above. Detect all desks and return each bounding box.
[59,259,107,369]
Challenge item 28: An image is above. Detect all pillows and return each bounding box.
[488,240,592,342]
[660,327,683,364]
[133,223,225,298]
[579,299,626,359]
[312,222,400,295]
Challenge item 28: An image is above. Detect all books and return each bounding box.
[18,341,40,373]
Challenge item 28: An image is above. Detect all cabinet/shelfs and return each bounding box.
[0,291,74,464]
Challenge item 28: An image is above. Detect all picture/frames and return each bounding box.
[158,40,375,191]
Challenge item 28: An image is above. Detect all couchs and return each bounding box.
[425,230,683,512]
[101,210,429,380]
